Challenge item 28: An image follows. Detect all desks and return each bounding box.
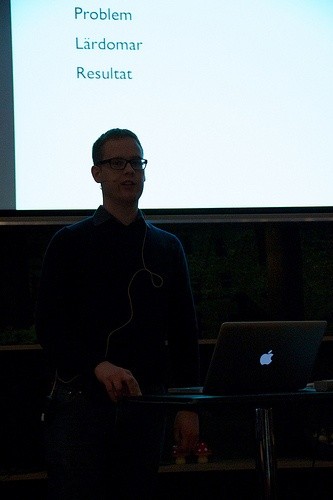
[117,384,333,500]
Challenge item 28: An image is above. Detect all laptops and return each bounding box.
[167,320,327,393]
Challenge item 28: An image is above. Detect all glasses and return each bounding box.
[95,157,147,171]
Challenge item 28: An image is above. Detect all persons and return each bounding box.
[32,129,201,500]
